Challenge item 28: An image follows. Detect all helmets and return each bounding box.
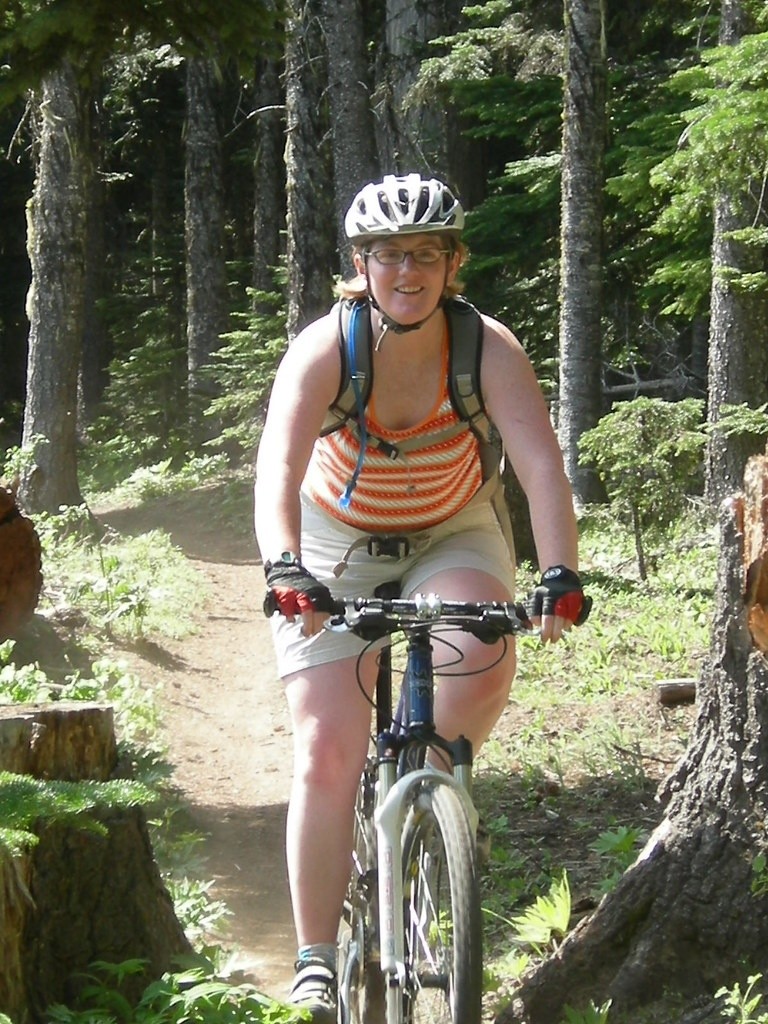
[345,173,465,240]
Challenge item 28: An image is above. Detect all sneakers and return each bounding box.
[431,822,490,865]
[288,955,338,1024]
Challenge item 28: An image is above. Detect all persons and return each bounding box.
[254,170,587,1024]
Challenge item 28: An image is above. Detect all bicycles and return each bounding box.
[263,590,596,1024]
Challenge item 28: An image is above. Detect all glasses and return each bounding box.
[361,247,455,265]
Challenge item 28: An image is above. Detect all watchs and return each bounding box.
[264,551,302,575]
